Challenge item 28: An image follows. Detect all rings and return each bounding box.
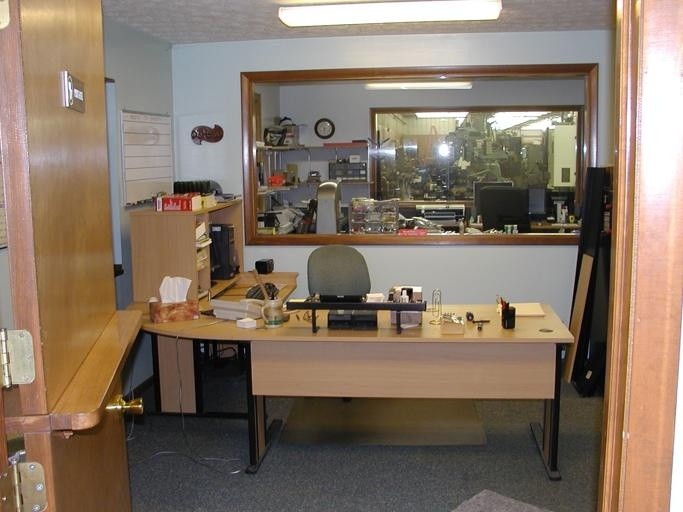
[496,297,509,328]
[296,314,300,319]
[473,320,491,322]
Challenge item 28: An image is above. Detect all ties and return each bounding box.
[473,180,515,223]
[479,185,531,233]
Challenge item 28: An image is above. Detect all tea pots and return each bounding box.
[241,64,598,245]
[370,105,583,208]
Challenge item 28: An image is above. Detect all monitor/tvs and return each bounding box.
[498,302,546,317]
[152,191,202,212]
[194,219,212,271]
[200,192,218,210]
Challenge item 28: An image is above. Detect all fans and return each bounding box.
[120,110,174,207]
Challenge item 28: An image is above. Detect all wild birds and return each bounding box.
[528,185,547,218]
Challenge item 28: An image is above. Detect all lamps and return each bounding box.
[307,245,371,403]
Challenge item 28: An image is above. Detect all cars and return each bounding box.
[261,297,283,329]
[502,308,515,328]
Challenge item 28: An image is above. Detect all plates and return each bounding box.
[257,146,369,235]
[129,200,244,303]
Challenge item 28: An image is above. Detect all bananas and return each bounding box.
[245,282,279,301]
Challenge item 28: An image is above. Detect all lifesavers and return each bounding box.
[208,223,238,281]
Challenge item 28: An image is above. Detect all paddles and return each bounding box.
[277,398,488,446]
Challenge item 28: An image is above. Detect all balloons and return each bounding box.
[314,118,335,139]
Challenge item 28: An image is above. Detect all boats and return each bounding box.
[254,258,275,274]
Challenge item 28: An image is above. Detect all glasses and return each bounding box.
[365,82,473,90]
[278,0,502,28]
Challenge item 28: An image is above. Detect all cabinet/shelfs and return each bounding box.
[399,289,409,303]
[458,219,464,235]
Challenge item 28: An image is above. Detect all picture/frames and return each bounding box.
[530,221,560,230]
[126,272,575,480]
